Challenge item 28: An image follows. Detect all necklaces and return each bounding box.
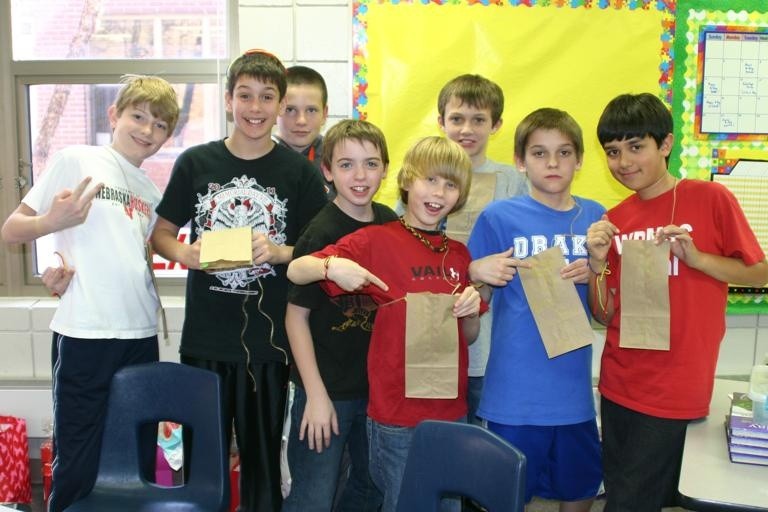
[398,214,449,254]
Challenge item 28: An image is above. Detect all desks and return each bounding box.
[677,378,768,512]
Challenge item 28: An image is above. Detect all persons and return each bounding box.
[286,137,488,511]
[1,72,178,512]
[465,107,607,511]
[151,49,330,511]
[396,75,528,426]
[269,66,339,197]
[584,93,768,511]
[282,119,395,511]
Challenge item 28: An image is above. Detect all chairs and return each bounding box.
[62,362,231,512]
[395,420,527,512]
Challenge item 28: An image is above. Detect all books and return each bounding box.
[723,391,768,466]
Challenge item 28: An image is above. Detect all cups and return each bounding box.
[746,363,768,431]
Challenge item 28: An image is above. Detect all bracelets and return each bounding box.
[323,252,336,282]
[587,256,614,322]
